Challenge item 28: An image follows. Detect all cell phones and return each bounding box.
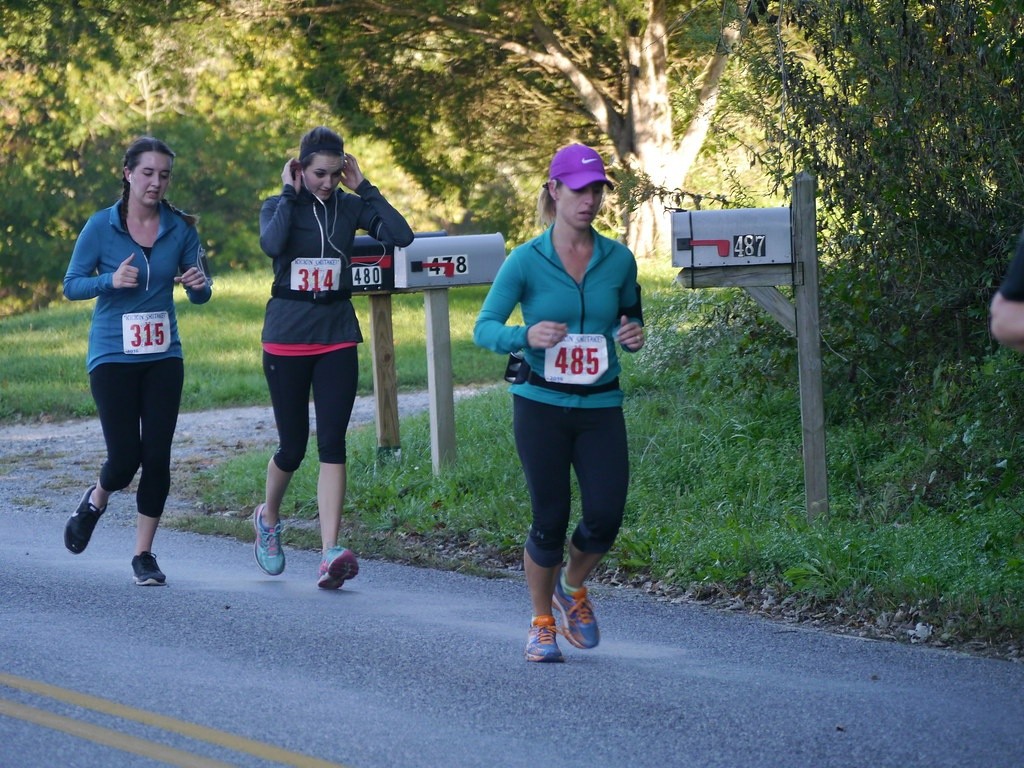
[199,252,213,286]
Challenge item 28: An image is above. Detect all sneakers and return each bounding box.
[131,551,167,586]
[315,544,358,590]
[551,573,599,650]
[64,485,107,554]
[253,502,286,577]
[525,614,563,662]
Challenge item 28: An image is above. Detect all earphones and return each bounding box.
[128,173,132,182]
[301,169,304,176]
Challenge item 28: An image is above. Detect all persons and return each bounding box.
[64,138,212,585]
[991,235,1024,352]
[253,128,414,589]
[473,143,645,661]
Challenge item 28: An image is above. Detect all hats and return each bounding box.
[550,142,615,192]
